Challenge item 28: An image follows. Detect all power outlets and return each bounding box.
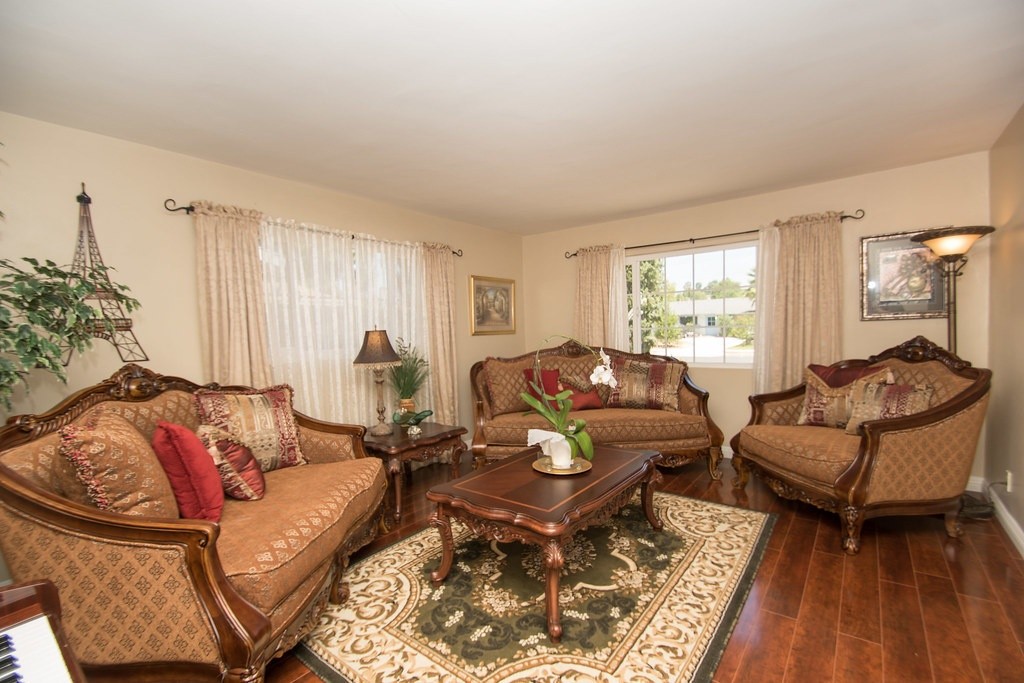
[1004,470,1012,492]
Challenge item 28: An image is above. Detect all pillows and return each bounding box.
[56,407,179,519]
[524,369,606,415]
[196,385,306,473]
[598,356,686,410]
[844,380,936,435]
[796,363,896,427]
[484,355,534,418]
[155,421,227,522]
[198,426,266,500]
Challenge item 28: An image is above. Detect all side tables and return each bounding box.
[362,423,467,524]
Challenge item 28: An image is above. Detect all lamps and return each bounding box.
[353,325,401,437]
[911,225,996,354]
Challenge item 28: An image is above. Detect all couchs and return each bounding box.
[0,363,396,683]
[469,339,725,480]
[730,335,993,554]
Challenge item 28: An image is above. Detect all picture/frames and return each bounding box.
[858,230,947,321]
[470,274,517,336]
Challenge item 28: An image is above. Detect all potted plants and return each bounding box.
[526,361,594,464]
[390,338,430,429]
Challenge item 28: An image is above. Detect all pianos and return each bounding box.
[0,577,93,683]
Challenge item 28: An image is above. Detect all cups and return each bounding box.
[536,451,552,471]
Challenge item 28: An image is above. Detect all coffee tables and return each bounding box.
[433,437,666,641]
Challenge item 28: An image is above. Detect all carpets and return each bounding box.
[295,490,778,683]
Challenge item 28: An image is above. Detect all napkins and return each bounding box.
[525,427,575,471]
